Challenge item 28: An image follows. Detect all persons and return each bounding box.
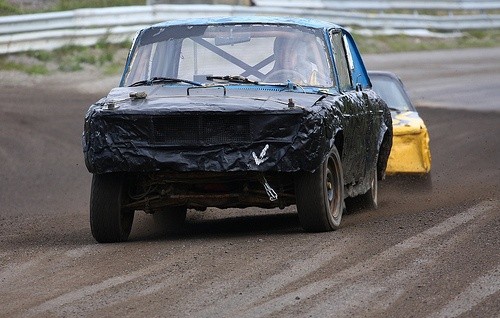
[264,36,321,87]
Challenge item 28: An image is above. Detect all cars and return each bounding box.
[84,16,393,243]
[365,70,432,175]
